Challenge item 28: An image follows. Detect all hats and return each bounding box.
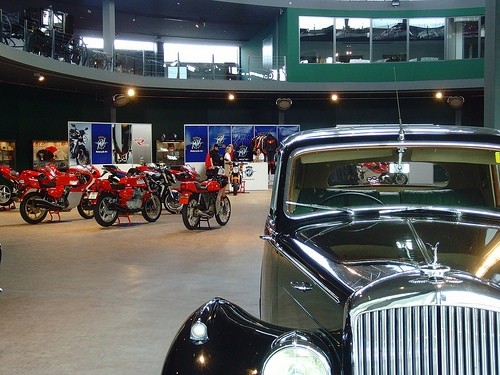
[214,144,220,147]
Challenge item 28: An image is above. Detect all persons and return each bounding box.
[252,148,265,162]
[205,143,239,193]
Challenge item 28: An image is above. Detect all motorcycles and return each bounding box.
[224,158,247,195]
[178,164,231,231]
[69,123,90,167]
[0,154,201,228]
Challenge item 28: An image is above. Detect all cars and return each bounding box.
[160,122,499,375]
